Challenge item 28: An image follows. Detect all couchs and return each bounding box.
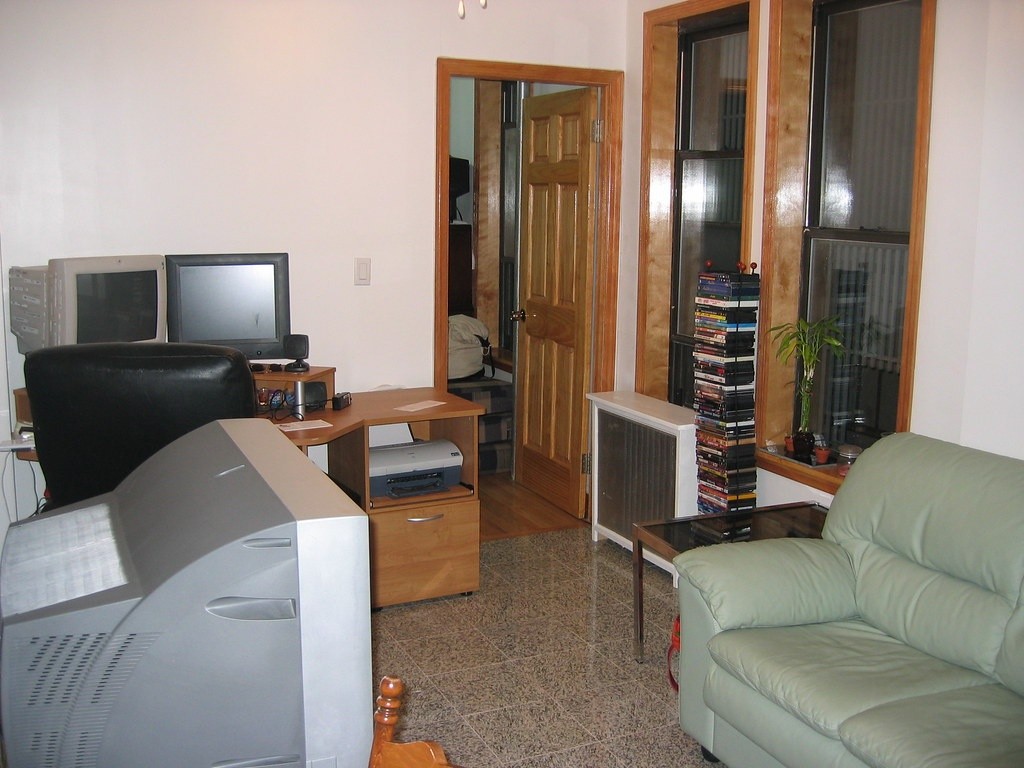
[672,431,1024,768]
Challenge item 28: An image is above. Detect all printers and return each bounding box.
[369,437,464,499]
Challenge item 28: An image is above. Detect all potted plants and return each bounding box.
[764,312,846,456]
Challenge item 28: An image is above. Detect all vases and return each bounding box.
[784,436,794,451]
[813,447,831,464]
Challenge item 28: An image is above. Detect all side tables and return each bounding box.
[632,500,830,664]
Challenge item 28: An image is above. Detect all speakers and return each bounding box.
[305,382,327,412]
[284,334,309,371]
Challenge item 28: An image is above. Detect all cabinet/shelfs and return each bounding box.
[9,367,487,617]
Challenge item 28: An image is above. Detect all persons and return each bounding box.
[448,293,490,382]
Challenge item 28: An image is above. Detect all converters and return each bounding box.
[332,392,352,411]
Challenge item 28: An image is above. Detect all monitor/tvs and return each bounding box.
[163,254,291,359]
[0,419,374,768]
[9,255,167,355]
